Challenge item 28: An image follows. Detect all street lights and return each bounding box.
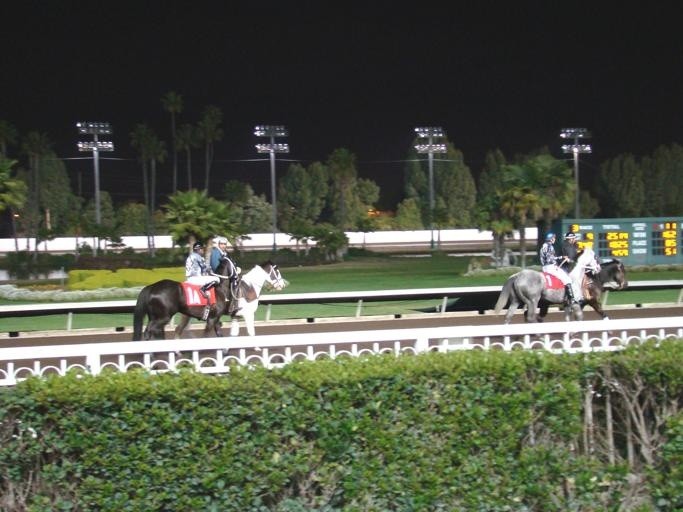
[254,126,290,250]
[76,121,114,224]
[414,127,447,248]
[560,126,592,219]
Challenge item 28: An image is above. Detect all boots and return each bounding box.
[199,280,219,298]
[564,283,578,305]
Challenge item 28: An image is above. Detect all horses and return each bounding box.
[516,258,628,341]
[494,247,602,352]
[172,259,287,356]
[132,250,243,370]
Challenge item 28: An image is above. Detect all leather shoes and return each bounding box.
[228,306,243,313]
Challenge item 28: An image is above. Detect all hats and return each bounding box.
[218,239,227,244]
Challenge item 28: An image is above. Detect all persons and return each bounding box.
[184,242,220,298]
[538,231,580,305]
[560,232,592,300]
[209,232,243,319]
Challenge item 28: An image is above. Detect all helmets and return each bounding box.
[545,233,556,241]
[192,242,206,251]
[564,232,576,239]
